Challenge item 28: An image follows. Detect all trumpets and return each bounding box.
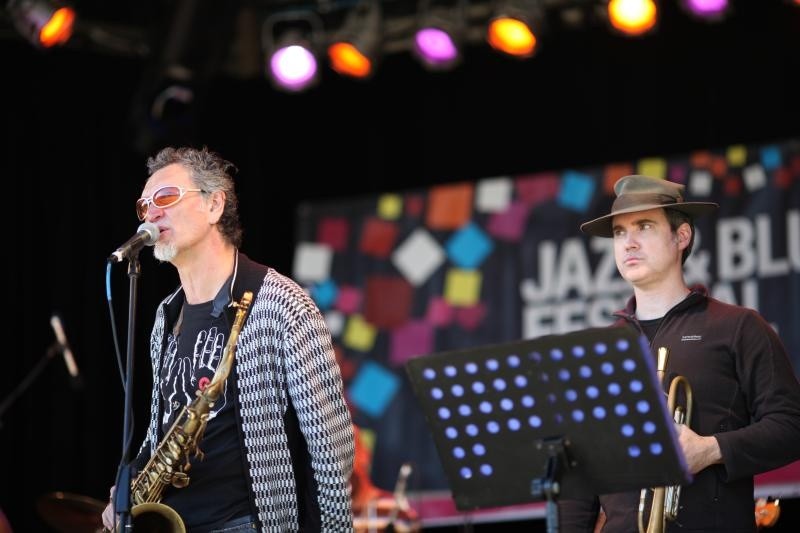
[636,345,695,532]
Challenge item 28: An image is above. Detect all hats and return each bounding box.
[579,175,718,237]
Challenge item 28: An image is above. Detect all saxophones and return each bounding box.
[101,289,256,533]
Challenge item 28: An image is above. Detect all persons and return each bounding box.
[102,146,354,533]
[559,175,800,533]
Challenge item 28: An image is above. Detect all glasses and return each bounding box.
[136,186,212,221]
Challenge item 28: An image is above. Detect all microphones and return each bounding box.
[106,223,160,266]
[49,315,79,376]
